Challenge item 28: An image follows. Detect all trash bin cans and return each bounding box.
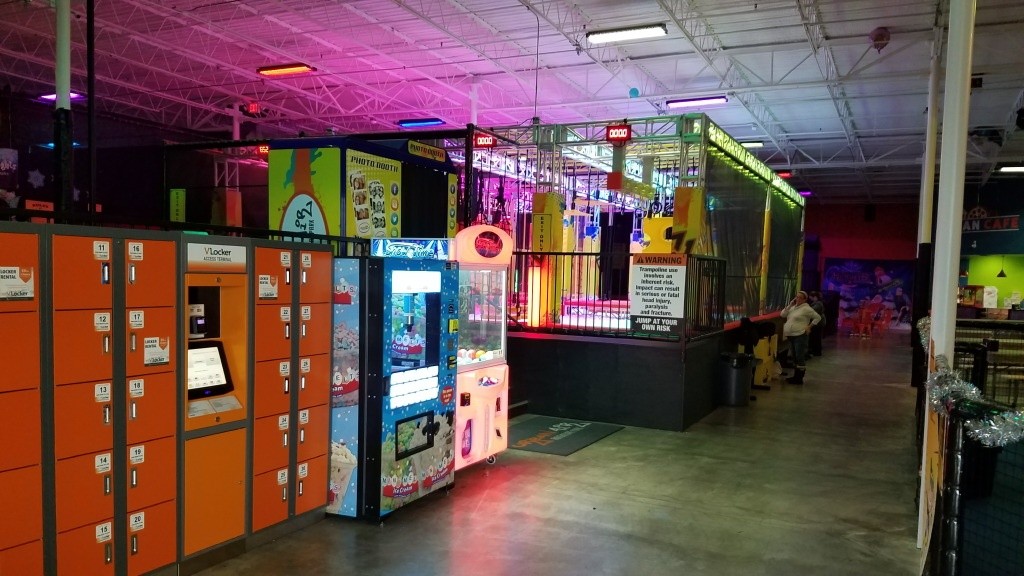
[719,351,754,407]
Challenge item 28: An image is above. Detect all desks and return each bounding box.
[955,329,1024,410]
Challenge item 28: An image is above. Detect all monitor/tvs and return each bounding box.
[188,347,226,391]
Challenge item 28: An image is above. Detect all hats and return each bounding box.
[799,291,808,300]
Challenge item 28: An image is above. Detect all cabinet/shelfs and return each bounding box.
[0,218,335,574]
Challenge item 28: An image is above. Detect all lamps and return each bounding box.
[740,141,764,148]
[996,255,1006,277]
[775,170,791,179]
[586,23,669,44]
[255,63,317,78]
[398,118,446,129]
[995,162,1024,173]
[666,96,728,109]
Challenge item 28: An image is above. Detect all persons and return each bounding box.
[807,291,826,357]
[780,290,822,384]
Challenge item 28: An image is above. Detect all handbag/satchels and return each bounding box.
[816,313,826,329]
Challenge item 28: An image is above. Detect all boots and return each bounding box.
[786,366,805,385]
